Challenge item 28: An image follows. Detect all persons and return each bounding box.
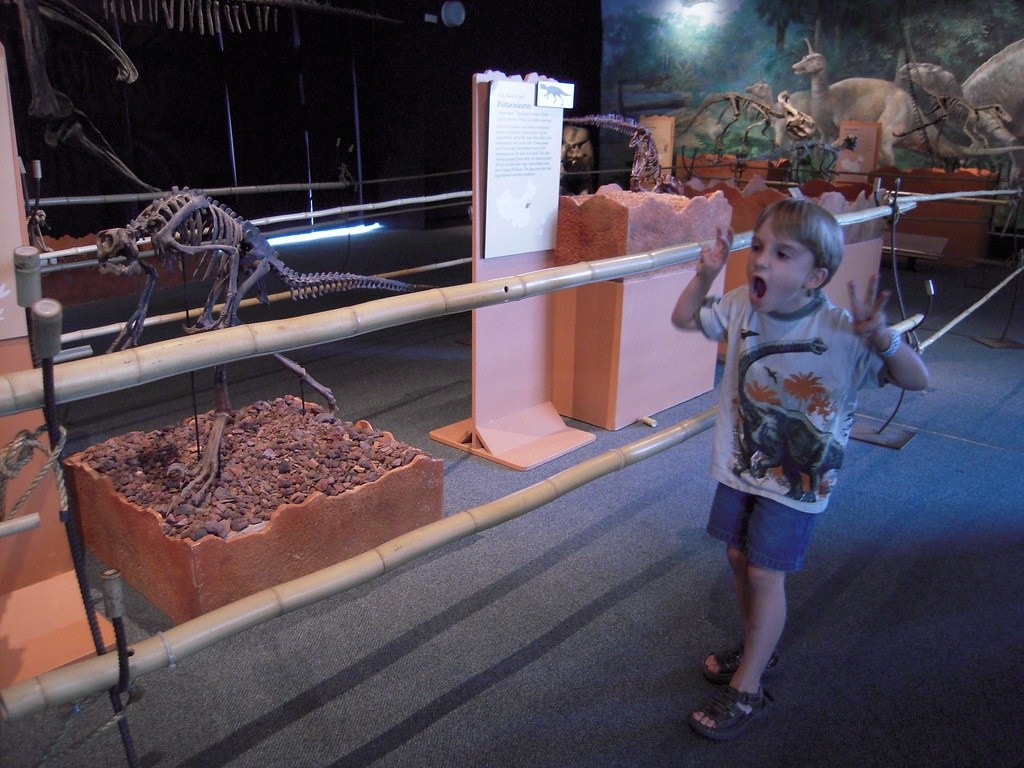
[671,200,929,744]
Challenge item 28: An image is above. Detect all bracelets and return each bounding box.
[872,329,902,361]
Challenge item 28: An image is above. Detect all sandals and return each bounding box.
[687,684,771,739]
[700,639,778,682]
